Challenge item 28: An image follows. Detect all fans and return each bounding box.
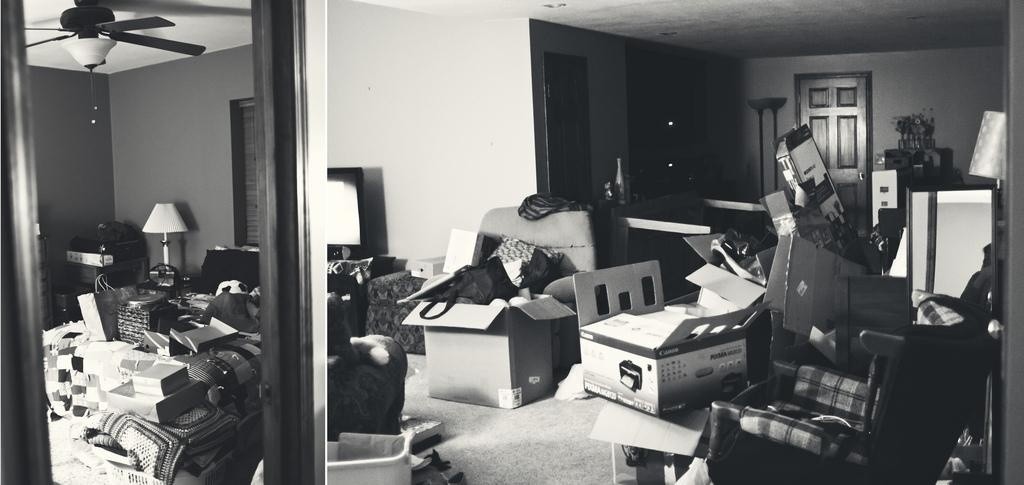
[24,0,206,57]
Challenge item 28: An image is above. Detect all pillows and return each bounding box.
[200,279,263,337]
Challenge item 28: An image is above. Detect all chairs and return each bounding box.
[465,206,598,362]
[327,290,407,440]
[709,289,995,485]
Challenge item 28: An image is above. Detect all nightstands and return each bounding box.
[137,278,193,300]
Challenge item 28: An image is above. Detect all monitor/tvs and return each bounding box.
[327,167,367,259]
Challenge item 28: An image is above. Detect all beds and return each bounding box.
[44,248,263,455]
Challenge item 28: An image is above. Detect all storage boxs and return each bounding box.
[589,394,714,485]
[117,302,176,343]
[400,286,576,410]
[573,260,765,417]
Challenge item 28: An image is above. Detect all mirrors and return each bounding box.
[905,182,999,484]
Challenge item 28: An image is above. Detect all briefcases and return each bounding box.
[117,302,179,351]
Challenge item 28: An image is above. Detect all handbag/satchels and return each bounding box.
[436,255,518,305]
[76,288,129,342]
[149,269,177,288]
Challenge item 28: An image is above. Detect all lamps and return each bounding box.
[143,203,189,271]
[61,32,117,123]
[968,111,1008,192]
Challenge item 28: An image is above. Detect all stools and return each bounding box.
[366,272,426,356]
